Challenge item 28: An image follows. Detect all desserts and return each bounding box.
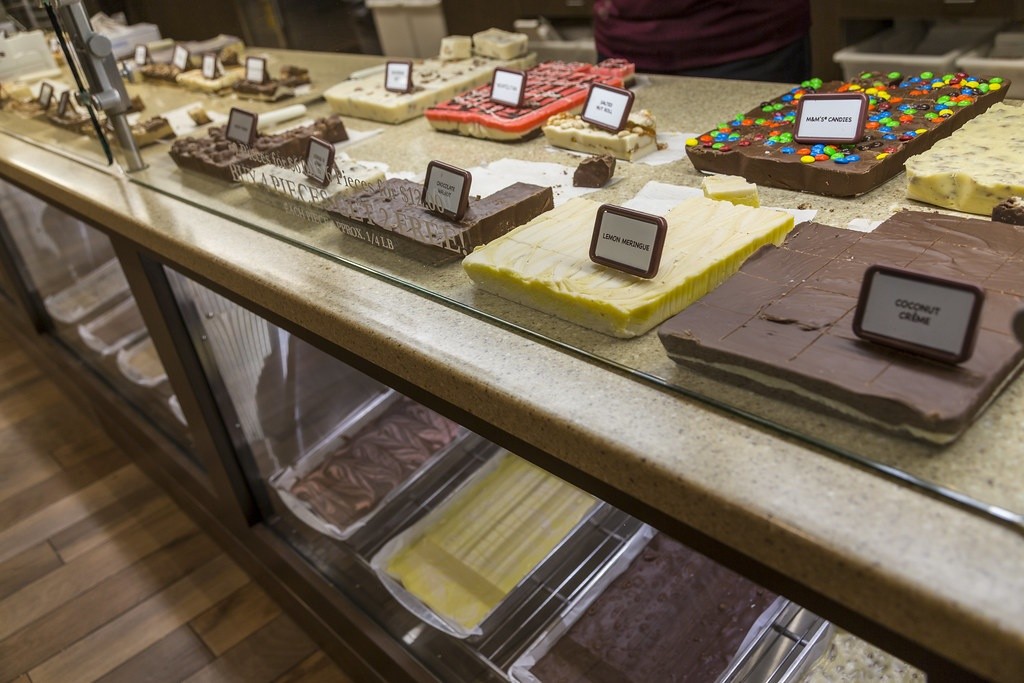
[0,29,1024,683]
